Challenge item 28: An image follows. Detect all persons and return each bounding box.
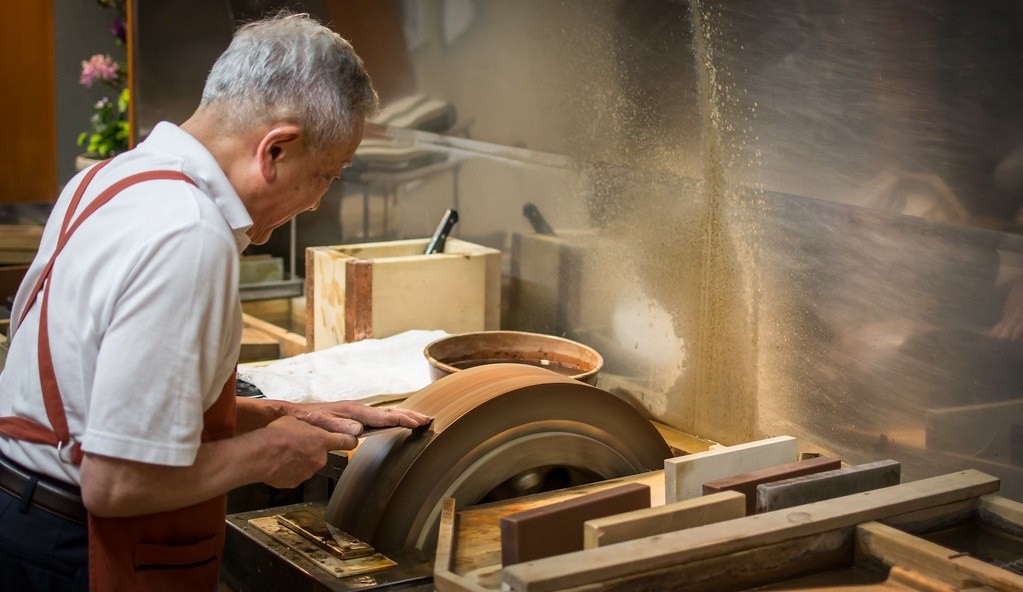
[0,15,430,592]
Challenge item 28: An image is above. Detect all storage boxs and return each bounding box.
[304,236,502,353]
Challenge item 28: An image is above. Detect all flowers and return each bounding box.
[76,0,144,160]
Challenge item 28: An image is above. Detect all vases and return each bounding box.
[75,152,105,170]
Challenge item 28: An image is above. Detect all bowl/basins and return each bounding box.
[423,331,604,387]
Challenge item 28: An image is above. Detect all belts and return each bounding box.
[0,460,87,525]
